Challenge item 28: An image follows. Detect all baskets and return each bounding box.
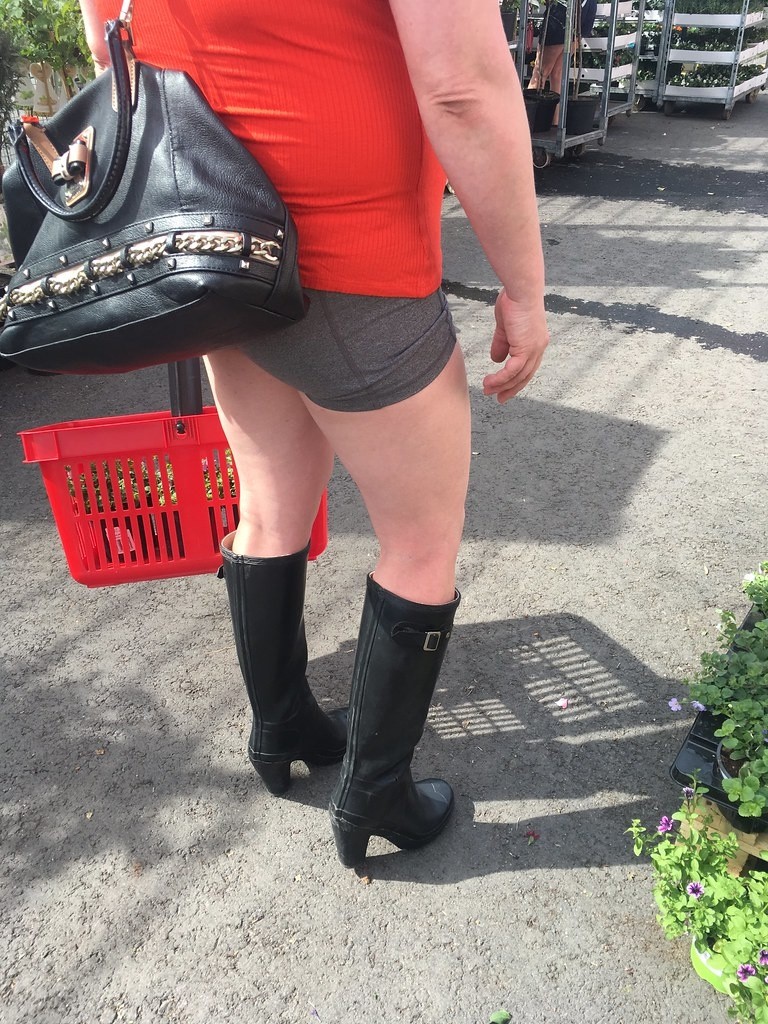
[18,404,326,592]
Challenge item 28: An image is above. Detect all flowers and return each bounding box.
[671,64,764,88]
[672,33,736,52]
[627,768,768,1024]
[669,562,768,818]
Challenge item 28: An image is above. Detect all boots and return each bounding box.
[326,574,454,870]
[214,532,352,796]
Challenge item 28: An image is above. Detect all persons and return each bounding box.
[580,0,598,38]
[78,0,547,869]
[527,0,565,126]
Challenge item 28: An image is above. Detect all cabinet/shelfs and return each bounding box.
[593,0,646,126]
[591,0,671,111]
[657,0,768,120]
[527,0,617,169]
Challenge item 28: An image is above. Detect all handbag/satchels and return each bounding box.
[1,0,309,377]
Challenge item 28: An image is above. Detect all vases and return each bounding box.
[669,46,758,63]
[665,82,744,98]
[691,935,730,995]
[668,604,768,833]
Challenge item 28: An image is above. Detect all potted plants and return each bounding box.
[498,0,521,42]
[524,0,560,131]
[568,0,666,90]
[674,0,768,27]
[727,26,768,86]
[566,0,600,136]
